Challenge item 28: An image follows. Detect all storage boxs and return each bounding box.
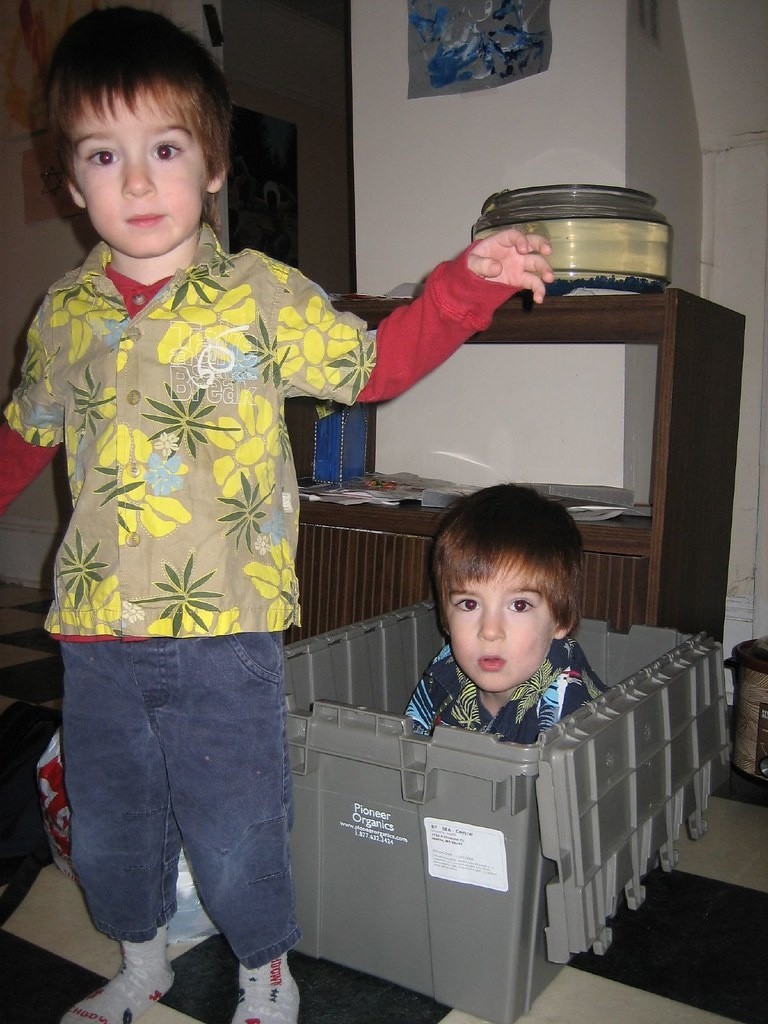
[283,601,732,1024]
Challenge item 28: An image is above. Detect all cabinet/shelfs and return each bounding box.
[281,287,747,644]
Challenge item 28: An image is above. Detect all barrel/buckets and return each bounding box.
[722,636,768,787]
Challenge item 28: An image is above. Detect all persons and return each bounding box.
[0,6,554,1024]
[405,484,611,744]
